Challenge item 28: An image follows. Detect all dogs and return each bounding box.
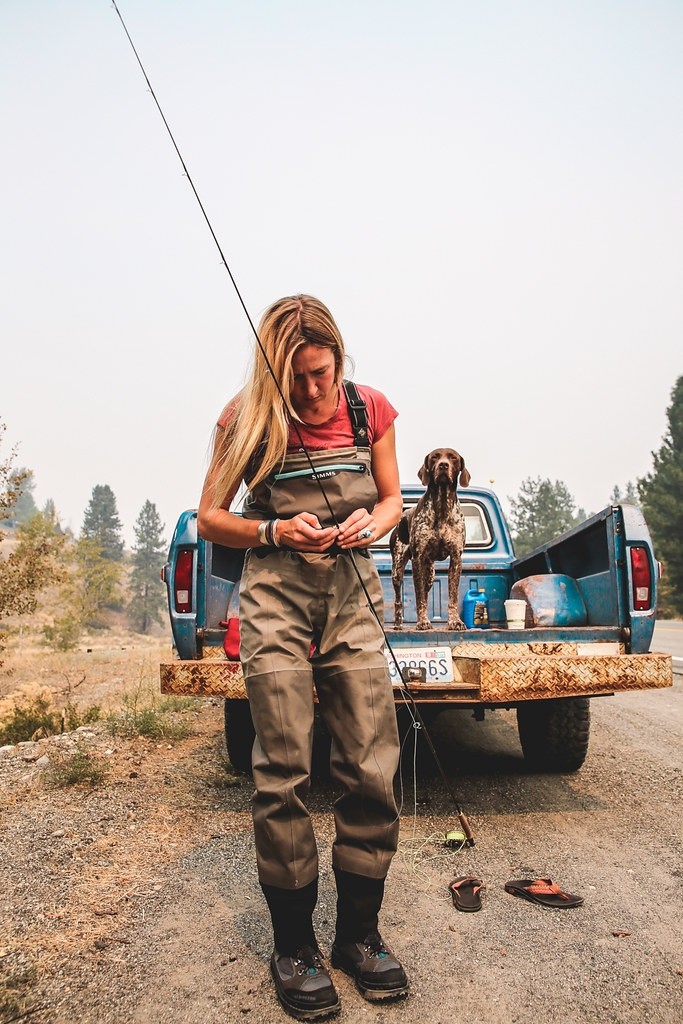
[388,448,471,631]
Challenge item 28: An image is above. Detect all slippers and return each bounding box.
[448,871,484,911]
[504,874,586,909]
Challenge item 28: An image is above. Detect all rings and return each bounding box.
[356,528,372,542]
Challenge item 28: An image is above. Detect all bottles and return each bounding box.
[462,588,490,629]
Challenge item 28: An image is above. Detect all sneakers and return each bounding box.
[330,928,410,1002]
[268,938,342,1022]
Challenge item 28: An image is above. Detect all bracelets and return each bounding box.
[253,515,282,550]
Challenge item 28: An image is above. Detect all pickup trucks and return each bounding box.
[158,486,671,771]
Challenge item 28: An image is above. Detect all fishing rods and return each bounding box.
[112,0,476,847]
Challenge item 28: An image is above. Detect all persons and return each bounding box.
[198,294,408,1019]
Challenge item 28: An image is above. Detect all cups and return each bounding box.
[504,599,527,629]
